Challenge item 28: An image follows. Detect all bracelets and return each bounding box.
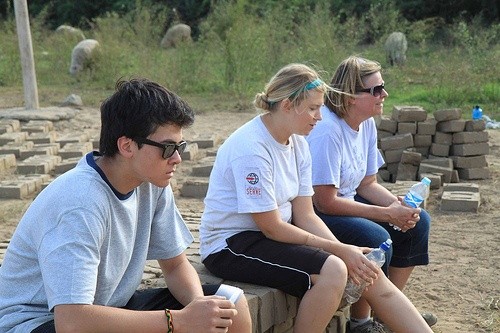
[164,307,174,333]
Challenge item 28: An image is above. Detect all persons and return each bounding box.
[196,62,436,333]
[304,56,438,333]
[0,78,253,333]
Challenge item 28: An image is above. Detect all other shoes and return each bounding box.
[372,312,438,332]
[345,319,385,333]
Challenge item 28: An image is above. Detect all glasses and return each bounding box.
[127,136,187,159]
[354,81,385,97]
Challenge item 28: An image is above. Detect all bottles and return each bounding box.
[389,177,431,231]
[343,238,393,304]
[472,105,482,120]
